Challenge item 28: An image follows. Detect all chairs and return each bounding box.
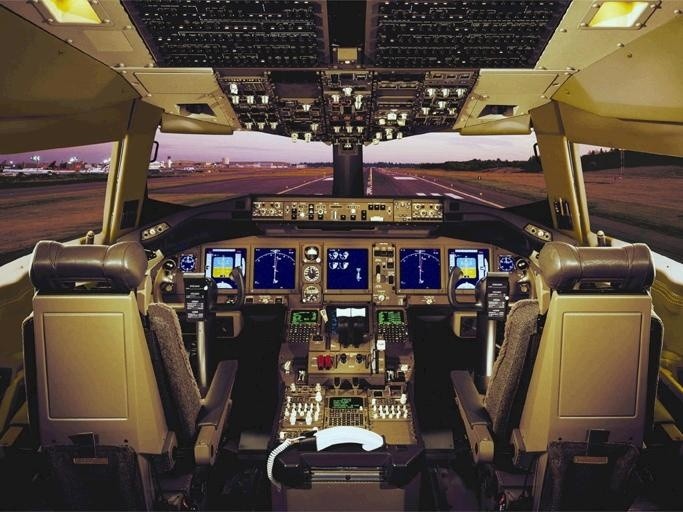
[450,242,682,512]
[1,240,238,512]
